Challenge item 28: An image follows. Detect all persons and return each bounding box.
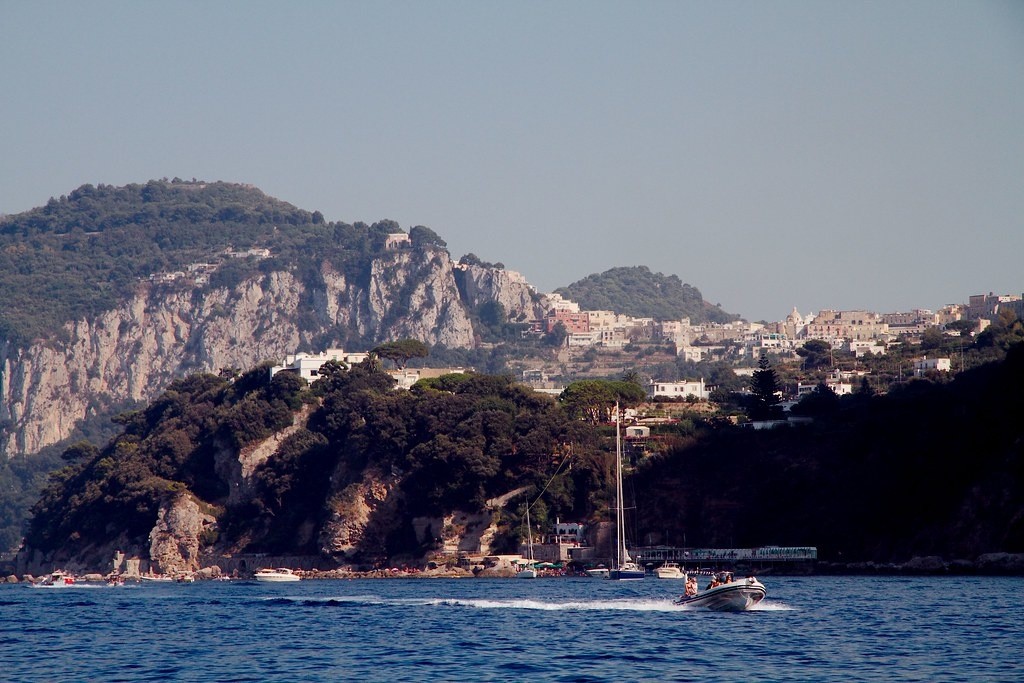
[685,571,732,596]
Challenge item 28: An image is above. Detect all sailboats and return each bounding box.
[516,505,538,579]
[606,399,648,580]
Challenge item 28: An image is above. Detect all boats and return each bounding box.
[670,575,768,612]
[586,569,609,579]
[253,568,302,581]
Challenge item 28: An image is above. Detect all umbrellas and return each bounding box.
[534,562,564,569]
[566,561,606,567]
